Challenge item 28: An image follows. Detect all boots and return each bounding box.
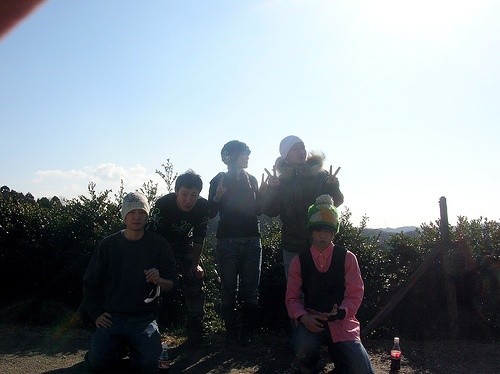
[238,303,257,352]
[225,305,240,345]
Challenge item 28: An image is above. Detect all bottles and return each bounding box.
[158,344,170,374]
[390,336,402,370]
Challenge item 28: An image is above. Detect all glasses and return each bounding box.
[312,227,336,233]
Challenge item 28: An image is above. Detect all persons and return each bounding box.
[284,194,373,374]
[147,174,213,350]
[82,192,180,374]
[208,139,270,347]
[266,135,344,280]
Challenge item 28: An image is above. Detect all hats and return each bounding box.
[121,191,150,223]
[278,135,304,159]
[221,140,251,167]
[303,194,340,233]
